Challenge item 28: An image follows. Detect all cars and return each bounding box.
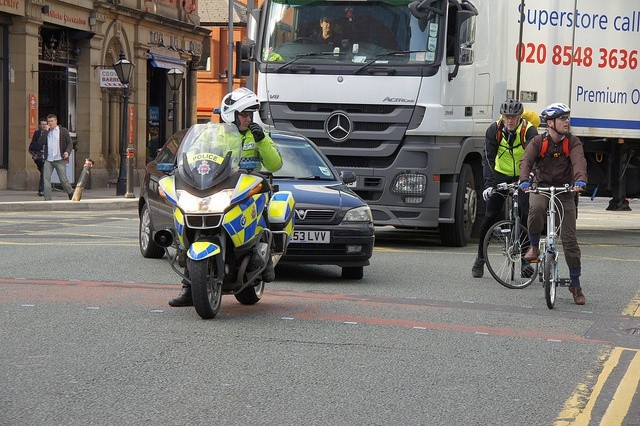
[138,128,376,280]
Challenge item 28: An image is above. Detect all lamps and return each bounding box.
[89,17,96,26]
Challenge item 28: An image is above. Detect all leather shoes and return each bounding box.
[168,284,194,307]
[262,253,275,283]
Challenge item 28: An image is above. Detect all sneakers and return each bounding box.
[522,265,534,279]
[524,246,542,260]
[568,284,586,304]
[472,256,486,278]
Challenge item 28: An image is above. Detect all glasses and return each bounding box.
[558,117,571,121]
[236,112,255,118]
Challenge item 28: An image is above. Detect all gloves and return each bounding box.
[574,180,586,188]
[519,180,530,190]
[482,187,495,202]
[250,124,265,141]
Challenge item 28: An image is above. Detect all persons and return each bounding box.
[471,98,539,278]
[149,124,154,150]
[28,118,48,197]
[168,87,283,306]
[519,103,588,306]
[153,124,160,150]
[44,113,75,199]
[307,13,345,43]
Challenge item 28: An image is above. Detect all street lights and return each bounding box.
[167,68,184,134]
[112,51,136,196]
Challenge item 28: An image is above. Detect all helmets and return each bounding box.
[541,103,570,119]
[500,98,523,115]
[221,87,260,126]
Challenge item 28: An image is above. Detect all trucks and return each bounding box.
[247,0,640,247]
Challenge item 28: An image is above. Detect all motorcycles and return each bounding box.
[154,123,296,319]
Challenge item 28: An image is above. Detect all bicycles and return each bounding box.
[482,182,538,289]
[518,186,587,309]
